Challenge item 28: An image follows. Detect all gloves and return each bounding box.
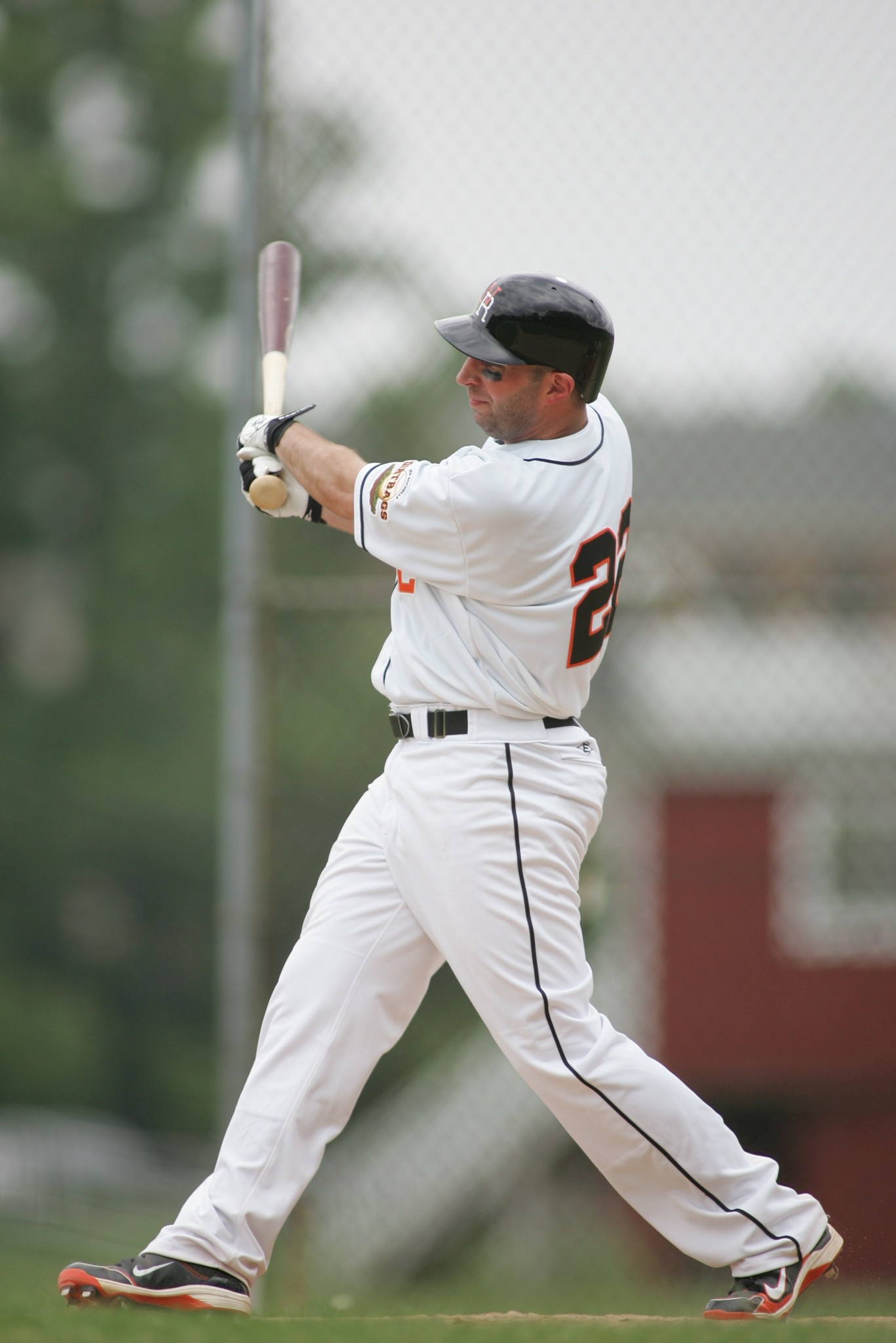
[236,404,316,454]
[236,447,327,524]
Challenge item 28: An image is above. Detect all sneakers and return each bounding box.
[58,1253,252,1319]
[703,1223,844,1320]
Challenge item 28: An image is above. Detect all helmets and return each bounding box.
[433,274,614,404]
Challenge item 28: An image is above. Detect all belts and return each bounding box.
[388,709,581,739]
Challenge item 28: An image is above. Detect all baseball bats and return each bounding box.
[249,240,301,509]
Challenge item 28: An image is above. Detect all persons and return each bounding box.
[56,274,844,1325]
